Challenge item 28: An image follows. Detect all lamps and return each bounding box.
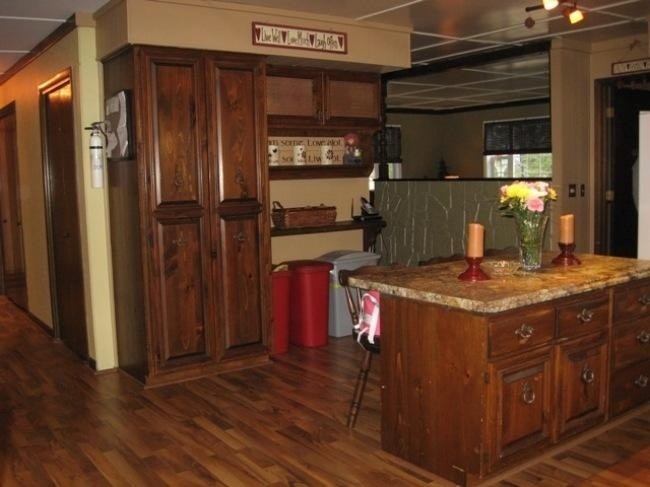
[568,10,584,25]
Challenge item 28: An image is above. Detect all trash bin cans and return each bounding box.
[272,264,292,356]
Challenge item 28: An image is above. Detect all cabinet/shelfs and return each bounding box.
[266,66,385,128]
[269,126,384,238]
[99,43,273,389]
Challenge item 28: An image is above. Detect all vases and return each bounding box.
[515,215,549,271]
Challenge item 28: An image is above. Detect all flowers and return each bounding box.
[496,182,557,217]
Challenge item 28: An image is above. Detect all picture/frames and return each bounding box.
[252,20,347,54]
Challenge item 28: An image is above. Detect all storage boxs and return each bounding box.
[273,272,289,354]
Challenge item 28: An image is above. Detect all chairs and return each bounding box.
[419,254,464,265]
[485,247,520,256]
[339,263,409,430]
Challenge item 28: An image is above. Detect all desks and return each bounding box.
[348,249,650,487]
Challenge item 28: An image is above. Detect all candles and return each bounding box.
[552,212,583,265]
[458,221,491,280]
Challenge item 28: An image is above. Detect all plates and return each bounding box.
[480,261,521,278]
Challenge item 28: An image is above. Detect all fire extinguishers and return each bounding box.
[84,121,108,190]
